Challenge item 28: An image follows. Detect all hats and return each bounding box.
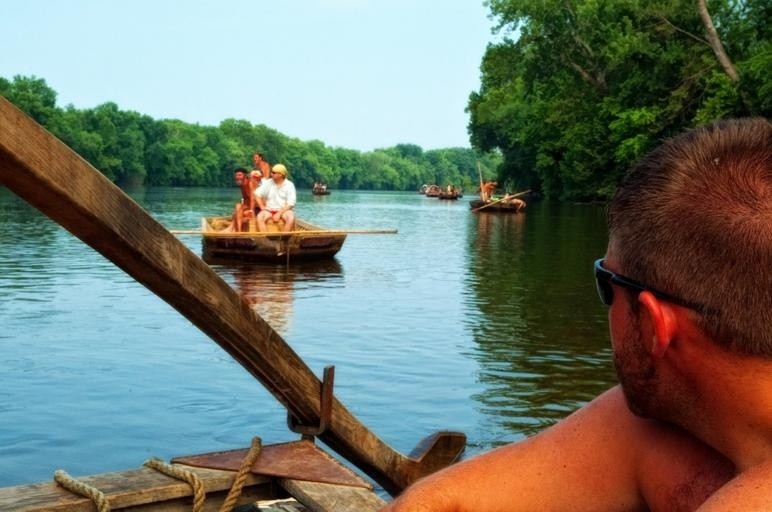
[272,164,288,176]
[235,168,248,174]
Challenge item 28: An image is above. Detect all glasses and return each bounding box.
[271,172,277,174]
[592,257,724,308]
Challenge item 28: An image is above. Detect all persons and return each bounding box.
[477,212,526,251]
[233,153,296,232]
[313,180,327,193]
[479,178,526,213]
[423,183,459,197]
[376,116,772,512]
[234,265,294,336]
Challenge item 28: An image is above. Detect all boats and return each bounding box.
[312,190,331,195]
[469,200,517,213]
[418,189,463,199]
[196,213,345,260]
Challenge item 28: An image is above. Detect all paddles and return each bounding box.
[0,94,467,502]
